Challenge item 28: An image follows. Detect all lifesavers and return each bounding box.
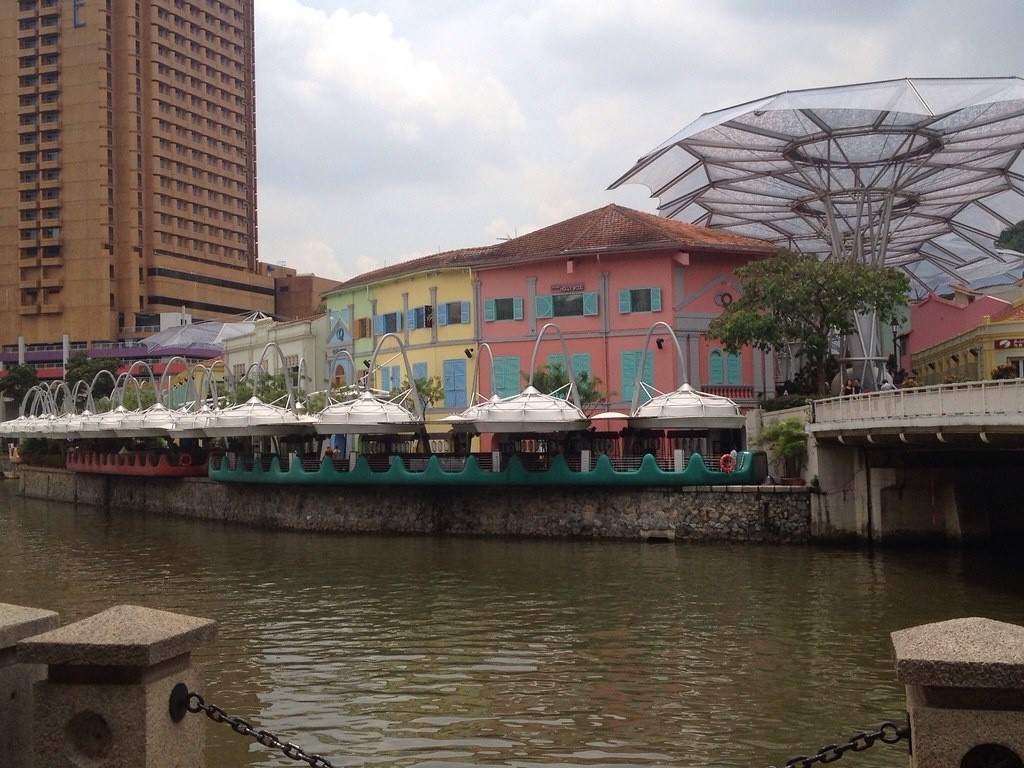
[719,454,737,472]
[180,453,192,466]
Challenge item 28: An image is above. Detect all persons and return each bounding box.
[904,374,919,394]
[840,379,863,400]
[881,380,898,396]
[730,446,737,458]
[325,445,333,458]
[332,446,340,459]
